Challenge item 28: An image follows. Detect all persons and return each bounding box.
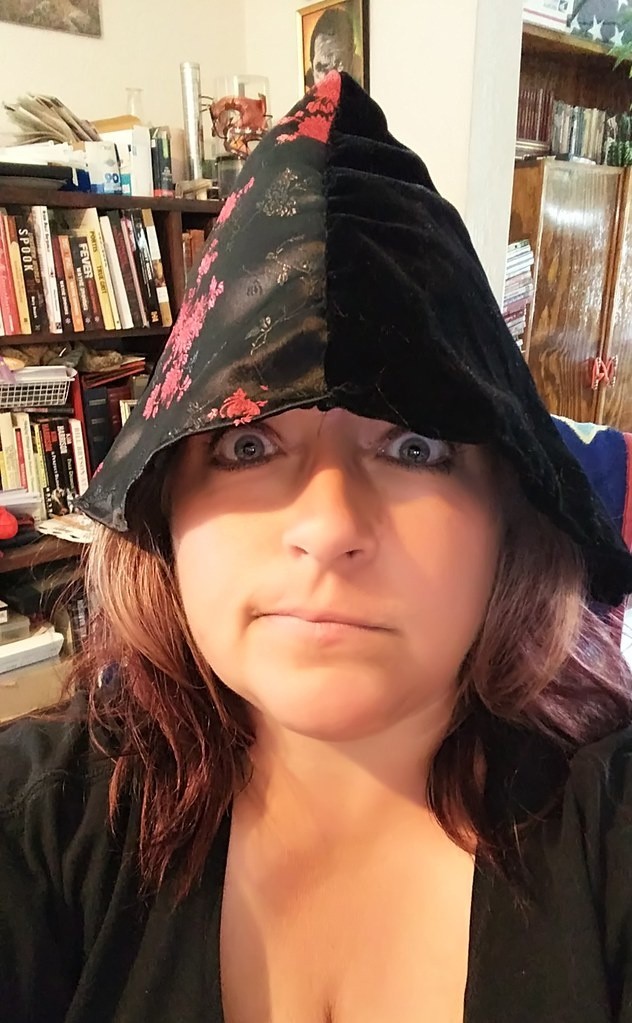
[0,68,632,1022]
[305,11,362,96]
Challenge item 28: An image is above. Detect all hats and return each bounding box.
[75,70,632,608]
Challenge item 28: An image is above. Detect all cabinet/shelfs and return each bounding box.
[508,159,632,437]
[1,181,224,732]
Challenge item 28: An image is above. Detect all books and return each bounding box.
[0,205,222,337]
[0,564,89,676]
[3,350,154,518]
[516,81,609,166]
[506,240,534,351]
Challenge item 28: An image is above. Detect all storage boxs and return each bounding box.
[2,607,75,722]
[1,114,173,199]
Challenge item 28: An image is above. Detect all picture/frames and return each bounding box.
[297,0,370,99]
